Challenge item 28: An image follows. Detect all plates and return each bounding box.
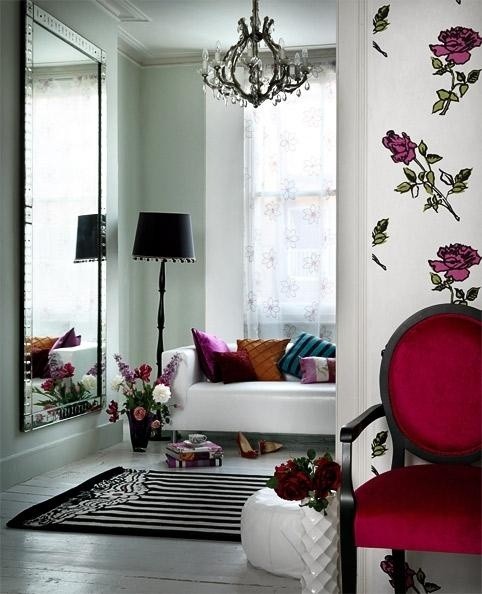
[184,439,207,446]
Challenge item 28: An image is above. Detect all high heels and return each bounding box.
[238,432,256,458]
[258,439,284,454]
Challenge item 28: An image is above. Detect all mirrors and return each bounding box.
[18,2,107,431]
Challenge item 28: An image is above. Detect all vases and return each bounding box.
[128,419,151,452]
[302,494,340,594]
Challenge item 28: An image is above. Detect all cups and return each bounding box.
[188,433,208,443]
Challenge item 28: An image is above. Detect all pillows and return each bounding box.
[212,351,258,384]
[192,328,230,383]
[277,332,336,379]
[27,328,81,380]
[237,339,290,381]
[298,355,336,384]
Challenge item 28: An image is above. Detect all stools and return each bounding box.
[241,489,304,579]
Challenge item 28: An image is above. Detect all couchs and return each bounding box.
[24,342,105,390]
[161,344,336,442]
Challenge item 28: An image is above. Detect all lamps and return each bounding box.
[132,212,197,441]
[197,2,311,107]
[74,214,107,262]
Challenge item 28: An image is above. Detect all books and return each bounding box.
[163,441,223,468]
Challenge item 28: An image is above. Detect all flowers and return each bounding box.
[429,242,481,305]
[379,549,440,593]
[107,355,182,436]
[382,131,471,223]
[267,450,341,508]
[34,364,99,415]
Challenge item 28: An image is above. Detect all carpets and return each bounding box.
[6,466,274,541]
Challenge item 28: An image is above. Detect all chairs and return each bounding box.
[341,303,480,593]
